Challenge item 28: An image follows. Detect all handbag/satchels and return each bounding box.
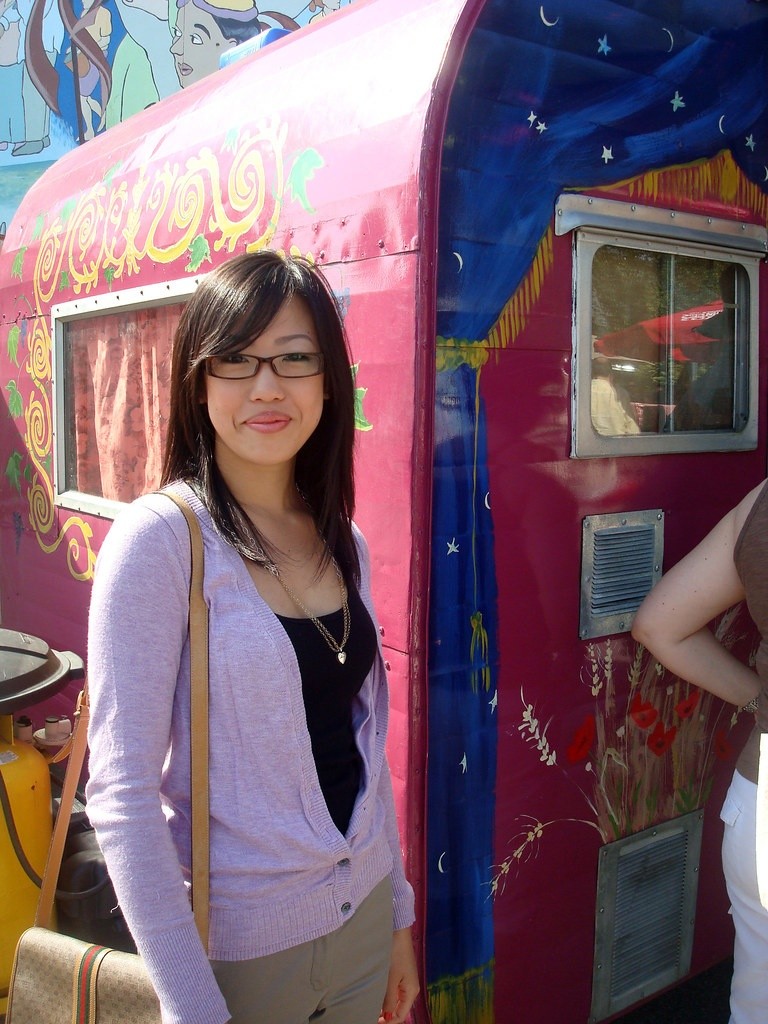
[4,926,162,1024]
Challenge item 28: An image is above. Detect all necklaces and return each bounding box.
[229,505,351,665]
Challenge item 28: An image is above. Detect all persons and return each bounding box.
[84,251,422,1024]
[590,356,642,435]
[632,478,768,1024]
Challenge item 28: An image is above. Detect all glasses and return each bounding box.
[200,351,326,380]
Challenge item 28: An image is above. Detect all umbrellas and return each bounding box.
[593,299,735,363]
